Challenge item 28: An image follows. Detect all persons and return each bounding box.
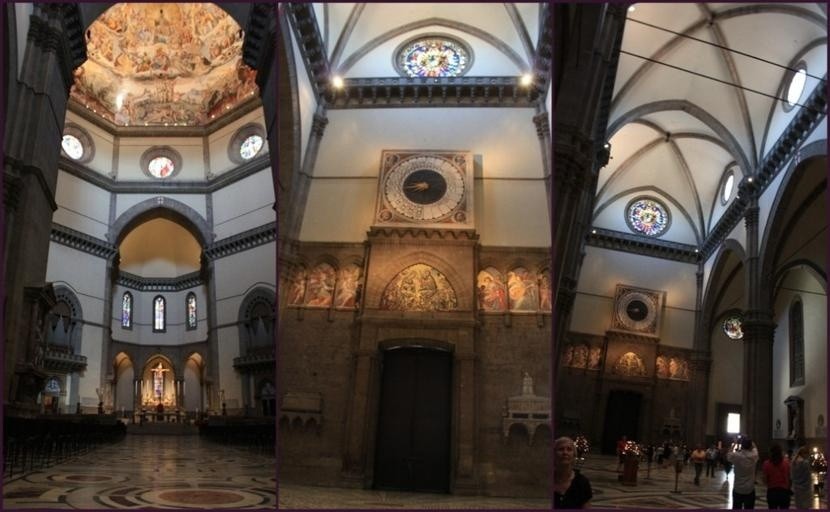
[553,436,593,510]
[97,402,105,415]
[615,434,816,511]
[77,402,83,415]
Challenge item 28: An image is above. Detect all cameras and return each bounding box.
[736,434,744,439]
